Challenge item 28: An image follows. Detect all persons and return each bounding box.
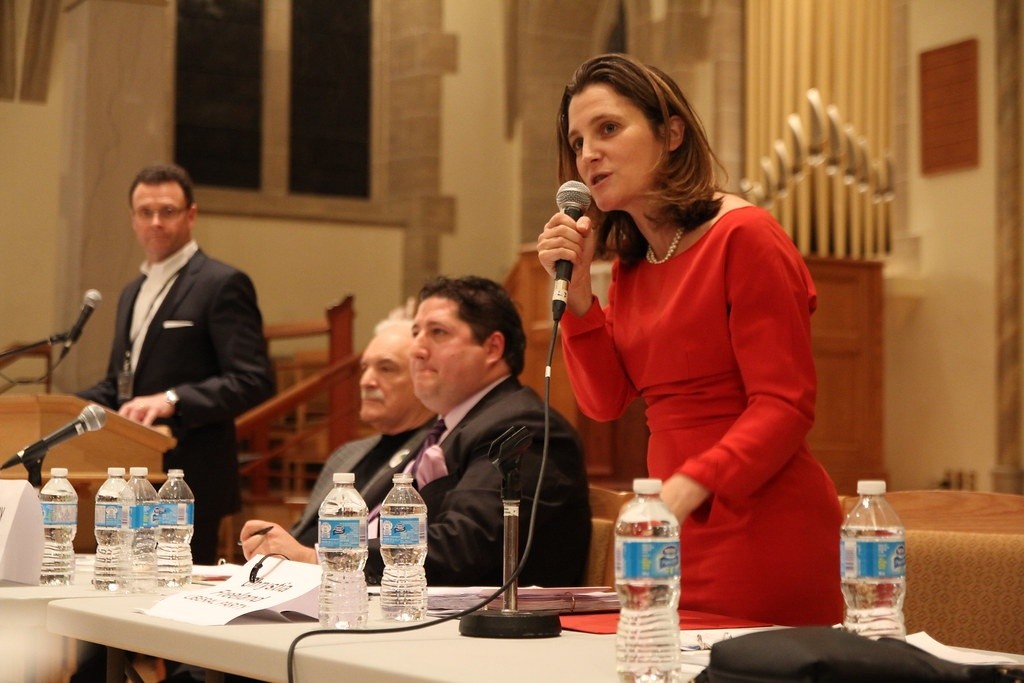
[286,320,443,567]
[73,165,278,562]
[537,53,846,624]
[241,274,589,585]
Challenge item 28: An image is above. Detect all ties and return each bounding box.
[369,419,447,526]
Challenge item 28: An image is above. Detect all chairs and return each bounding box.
[838,489,1024,655]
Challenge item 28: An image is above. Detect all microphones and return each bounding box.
[552,180,592,321]
[3,404,106,470]
[61,289,101,359]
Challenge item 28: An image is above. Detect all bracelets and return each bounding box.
[165,390,180,408]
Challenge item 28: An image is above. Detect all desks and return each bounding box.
[0,554,1024,683]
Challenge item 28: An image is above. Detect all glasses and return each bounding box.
[129,204,191,220]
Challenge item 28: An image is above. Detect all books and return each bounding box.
[425,584,619,615]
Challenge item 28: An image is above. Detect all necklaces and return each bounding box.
[644,228,682,266]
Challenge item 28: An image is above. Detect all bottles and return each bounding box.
[839,480,906,642]
[614,478,681,683]
[317,473,369,630]
[379,473,428,622]
[39,468,78,587]
[94,468,195,594]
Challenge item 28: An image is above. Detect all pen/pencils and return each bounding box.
[238,526,274,545]
